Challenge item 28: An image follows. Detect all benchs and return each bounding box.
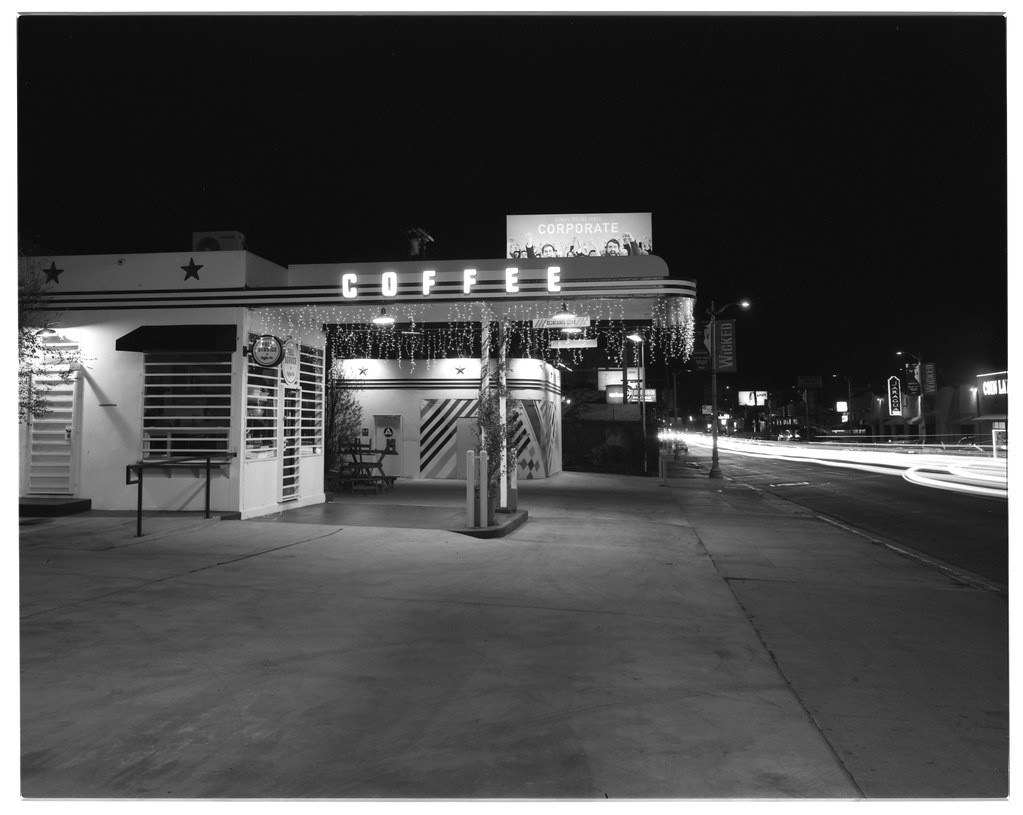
[326,474,400,497]
[338,437,400,456]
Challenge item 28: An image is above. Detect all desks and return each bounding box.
[345,465,389,493]
[348,438,389,466]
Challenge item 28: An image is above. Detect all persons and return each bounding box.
[996,432,1004,451]
[509,232,652,258]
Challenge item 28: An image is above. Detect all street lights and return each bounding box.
[896,350,924,444]
[674,369,691,433]
[626,332,647,473]
[706,298,751,479]
[792,385,809,441]
[833,374,853,435]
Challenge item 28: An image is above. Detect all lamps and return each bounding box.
[551,302,576,319]
[372,307,395,325]
[400,322,420,335]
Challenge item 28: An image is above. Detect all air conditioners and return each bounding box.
[192,231,247,252]
